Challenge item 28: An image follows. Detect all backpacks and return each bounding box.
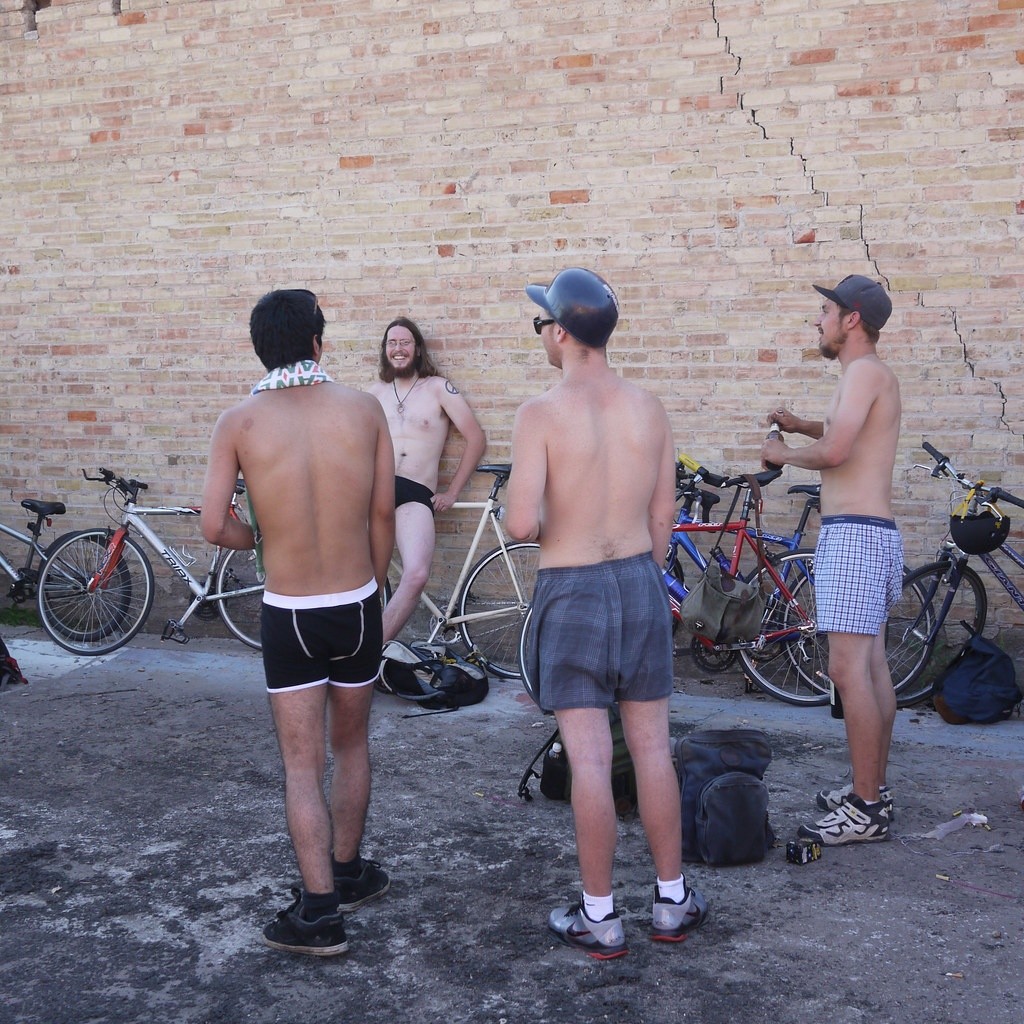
[0,636,29,692]
[516,694,645,808]
[377,637,490,719]
[929,619,1023,725]
[677,726,778,869]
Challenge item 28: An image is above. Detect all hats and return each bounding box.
[811,275,896,332]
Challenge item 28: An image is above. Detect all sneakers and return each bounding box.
[797,792,890,847]
[650,873,709,945]
[815,784,894,821]
[332,858,391,914]
[545,892,631,960]
[257,886,352,958]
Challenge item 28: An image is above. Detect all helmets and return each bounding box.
[524,265,620,347]
[947,479,1013,557]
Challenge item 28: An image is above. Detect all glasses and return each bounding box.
[384,339,414,348]
[294,287,321,329]
[531,315,557,335]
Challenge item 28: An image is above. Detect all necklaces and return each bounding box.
[393,377,420,412]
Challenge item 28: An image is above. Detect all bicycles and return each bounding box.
[506,456,937,723]
[1,499,132,643]
[374,464,544,682]
[32,466,264,652]
[886,442,1024,712]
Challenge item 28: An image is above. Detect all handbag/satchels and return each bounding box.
[677,563,766,644]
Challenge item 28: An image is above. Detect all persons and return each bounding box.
[505,268,707,959]
[760,275,904,848]
[200,288,396,956]
[366,318,487,645]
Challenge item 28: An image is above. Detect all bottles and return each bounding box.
[660,566,691,604]
[711,547,745,583]
[549,742,563,760]
[829,678,843,719]
[765,420,784,471]
